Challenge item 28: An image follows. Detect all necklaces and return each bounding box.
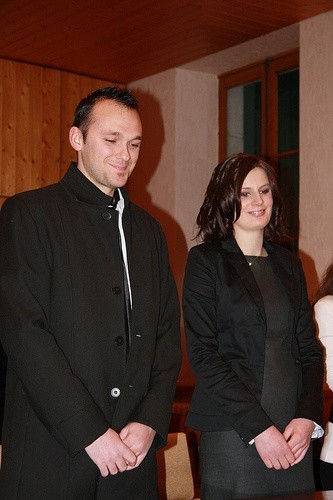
[244,251,260,266]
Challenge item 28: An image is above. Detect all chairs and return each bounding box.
[155,433,195,500]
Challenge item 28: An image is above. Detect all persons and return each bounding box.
[182,153,323,500]
[0,88,178,500]
[314,261,333,491]
